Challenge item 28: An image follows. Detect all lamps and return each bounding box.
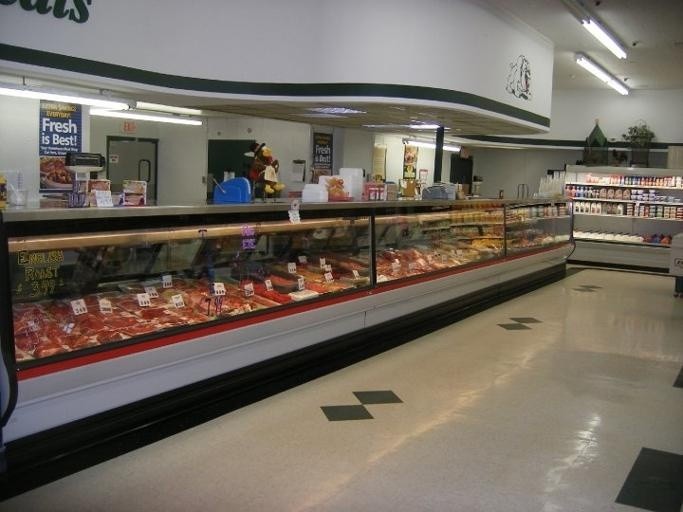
[563,0,630,95]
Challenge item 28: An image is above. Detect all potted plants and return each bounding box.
[622,119,656,168]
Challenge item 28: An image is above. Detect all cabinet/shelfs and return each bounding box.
[565,165,683,271]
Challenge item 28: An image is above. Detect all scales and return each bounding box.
[65,153,105,192]
